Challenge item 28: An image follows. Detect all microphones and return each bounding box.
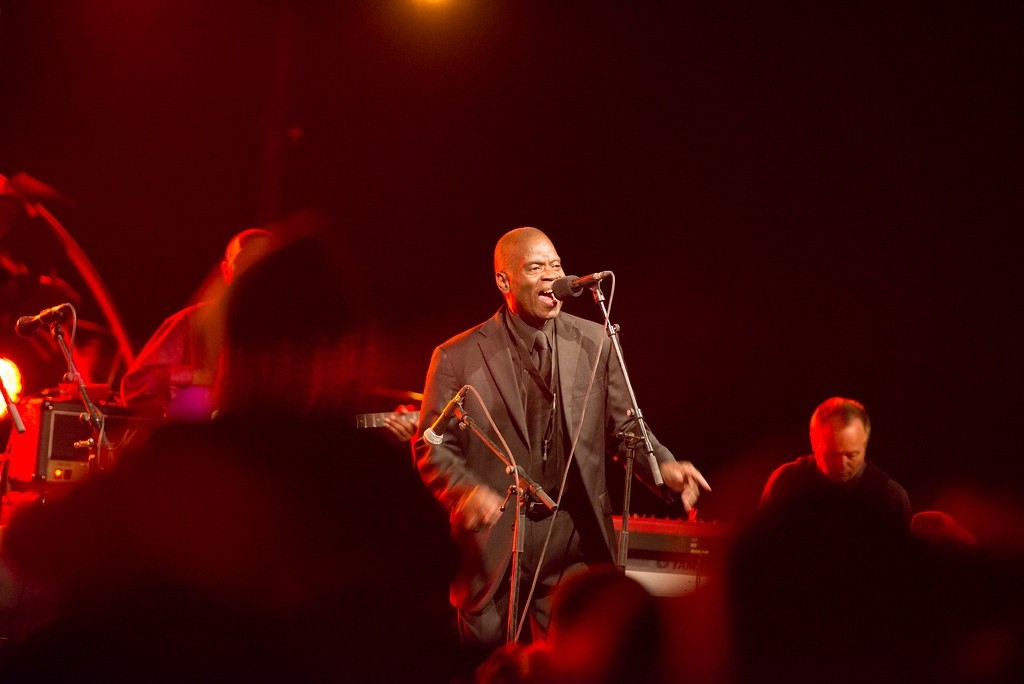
[552,270,609,301]
[16,304,69,337]
[422,385,467,445]
[8,172,72,209]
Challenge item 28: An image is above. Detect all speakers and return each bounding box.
[37,397,135,482]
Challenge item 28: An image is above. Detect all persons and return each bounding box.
[756,396,913,550]
[119,228,283,427]
[410,226,712,657]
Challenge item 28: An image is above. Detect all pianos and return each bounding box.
[612,513,732,599]
